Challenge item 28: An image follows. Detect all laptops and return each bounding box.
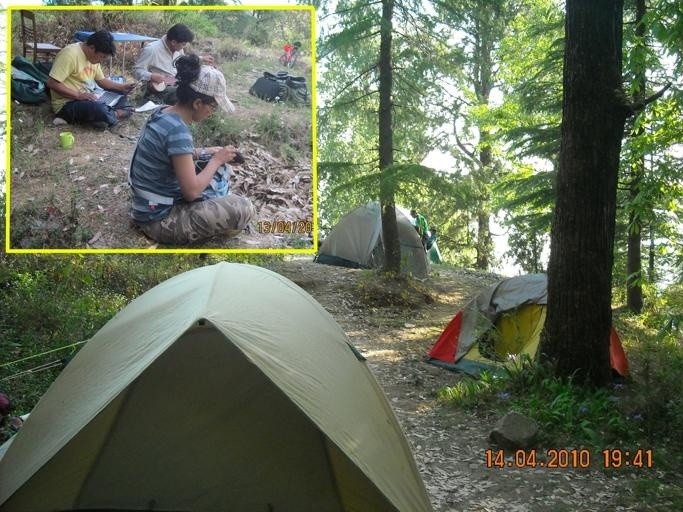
[90,75,143,109]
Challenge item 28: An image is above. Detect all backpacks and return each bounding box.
[11,65,48,103]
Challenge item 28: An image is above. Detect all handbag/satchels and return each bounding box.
[173,158,229,202]
[249,70,308,106]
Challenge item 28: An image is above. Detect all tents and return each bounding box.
[424,272,634,385]
[1,260,432,511]
[312,199,429,275]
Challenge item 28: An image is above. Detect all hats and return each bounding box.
[184,65,236,114]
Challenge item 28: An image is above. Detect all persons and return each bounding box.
[125,53,255,247]
[46,28,138,127]
[408,208,429,254]
[426,225,438,251]
[131,23,215,107]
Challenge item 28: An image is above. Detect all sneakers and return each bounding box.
[114,109,130,119]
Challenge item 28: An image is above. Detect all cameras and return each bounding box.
[228,150,245,164]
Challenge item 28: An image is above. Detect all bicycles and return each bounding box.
[279,40,302,68]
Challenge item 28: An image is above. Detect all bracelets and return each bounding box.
[200,146,208,161]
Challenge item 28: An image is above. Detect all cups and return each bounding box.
[60,131,74,149]
